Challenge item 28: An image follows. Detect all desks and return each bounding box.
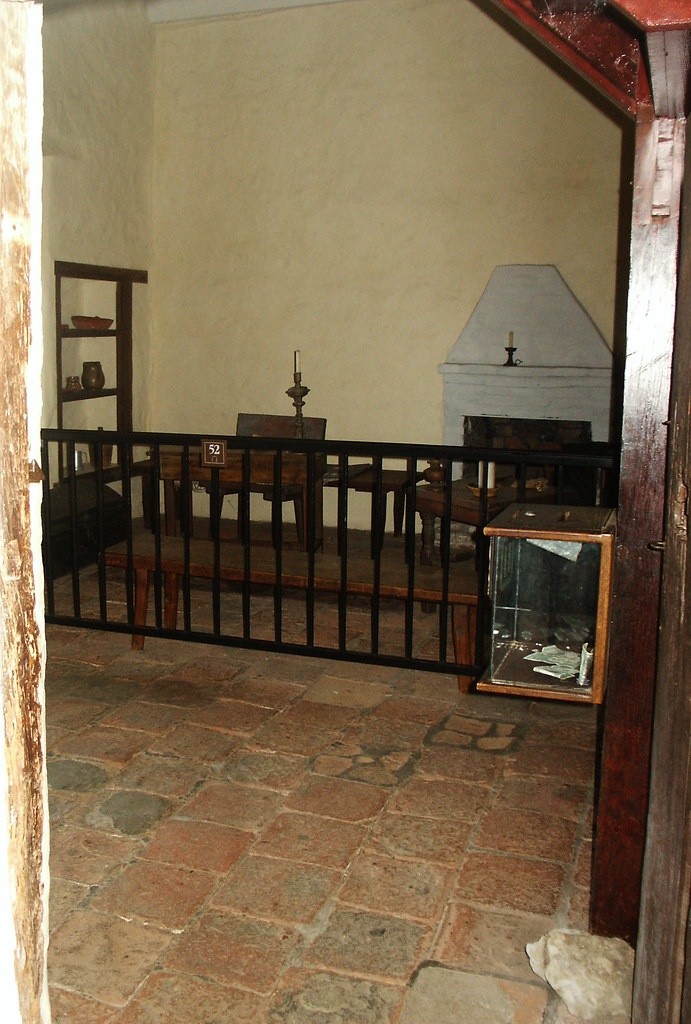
[415,480,559,614]
[145,444,325,556]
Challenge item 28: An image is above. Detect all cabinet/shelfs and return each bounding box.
[56,272,122,481]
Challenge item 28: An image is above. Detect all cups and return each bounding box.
[75,451,87,470]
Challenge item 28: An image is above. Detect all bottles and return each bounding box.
[89,427,112,466]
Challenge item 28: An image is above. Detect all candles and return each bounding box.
[295,349,301,373]
[478,461,495,489]
[509,331,513,347]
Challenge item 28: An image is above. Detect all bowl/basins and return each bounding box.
[72,316,113,329]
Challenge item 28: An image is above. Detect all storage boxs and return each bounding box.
[491,536,602,693]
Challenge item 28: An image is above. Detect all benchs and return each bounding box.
[98,532,478,693]
